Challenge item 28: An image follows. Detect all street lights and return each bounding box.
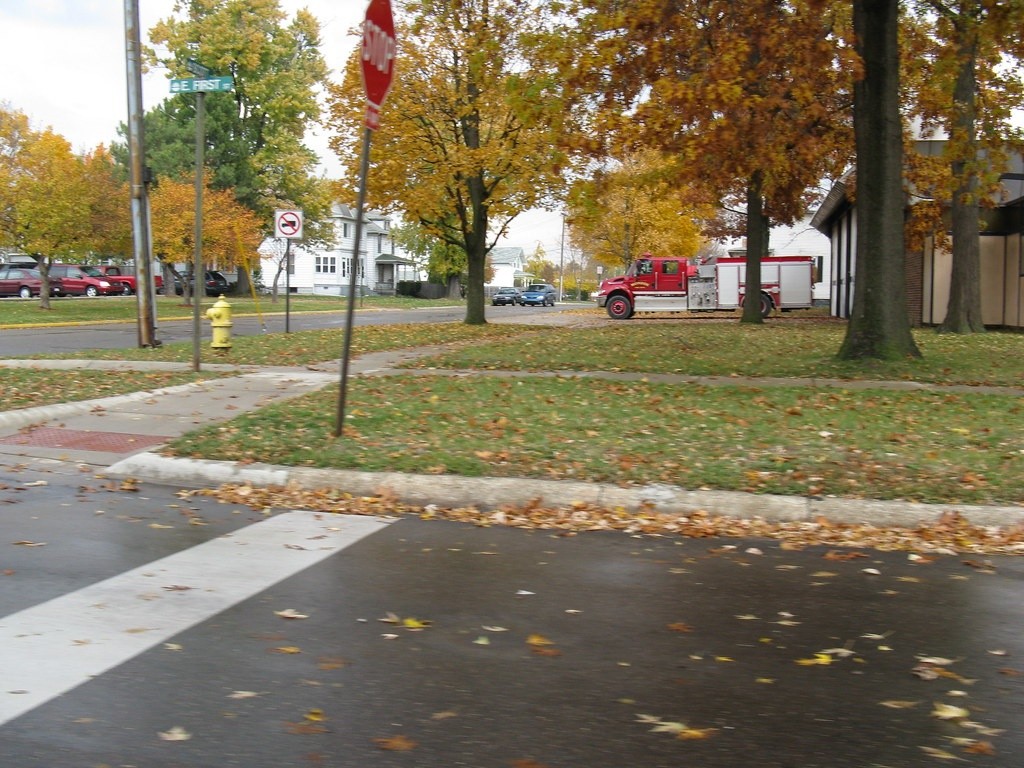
[559,212,569,301]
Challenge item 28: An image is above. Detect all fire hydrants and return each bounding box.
[206,293,235,353]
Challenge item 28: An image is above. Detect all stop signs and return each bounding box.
[357,1,398,132]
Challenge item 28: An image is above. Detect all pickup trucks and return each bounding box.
[88,265,163,296]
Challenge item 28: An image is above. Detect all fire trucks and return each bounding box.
[596,251,817,319]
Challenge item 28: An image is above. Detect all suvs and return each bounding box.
[32,263,125,296]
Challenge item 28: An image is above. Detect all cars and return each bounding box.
[159,270,228,297]
[521,283,557,307]
[1,268,65,298]
[492,288,522,306]
